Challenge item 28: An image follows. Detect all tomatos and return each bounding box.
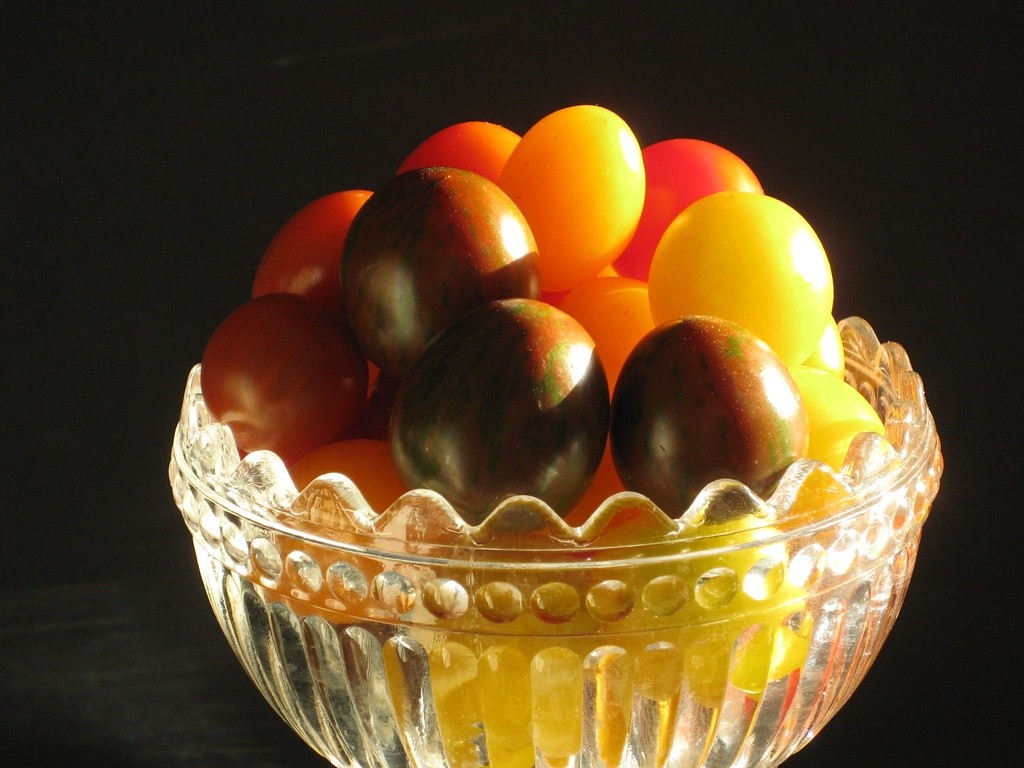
[200,105,890,620]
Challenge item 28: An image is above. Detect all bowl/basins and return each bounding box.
[169,317,943,768]
